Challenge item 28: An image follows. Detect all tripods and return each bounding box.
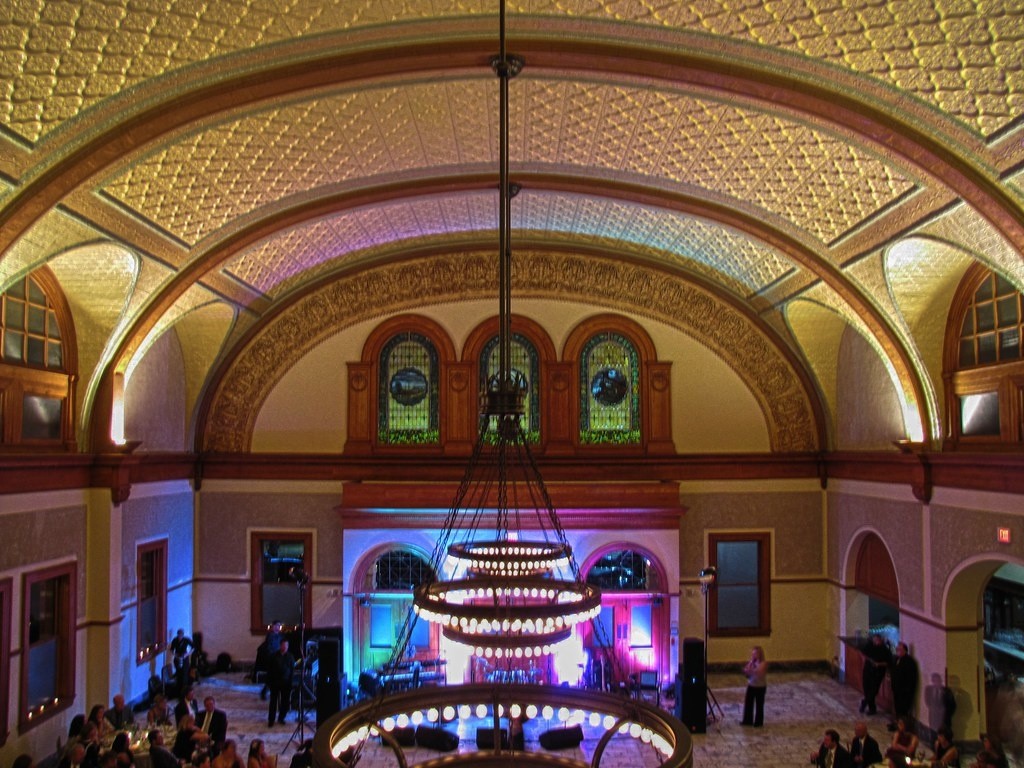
[280,574,316,754]
[703,582,724,722]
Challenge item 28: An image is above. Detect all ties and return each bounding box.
[826,750,832,768]
[203,712,211,732]
[859,738,863,755]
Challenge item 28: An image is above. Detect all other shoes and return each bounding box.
[866,704,877,715]
[740,722,751,725]
[269,720,274,727]
[279,720,286,724]
[859,700,867,712]
[753,723,764,727]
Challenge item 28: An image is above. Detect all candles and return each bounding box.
[154,644,159,652]
[40,705,45,715]
[53,698,59,709]
[28,712,32,722]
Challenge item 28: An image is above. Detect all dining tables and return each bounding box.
[97,728,177,768]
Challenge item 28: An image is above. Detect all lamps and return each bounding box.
[310,1,694,768]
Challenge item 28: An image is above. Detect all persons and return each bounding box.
[170,628,197,695]
[259,620,286,700]
[739,647,767,727]
[12,687,314,768]
[805,717,1009,768]
[923,673,957,756]
[886,641,918,731]
[266,639,295,727]
[346,682,360,708]
[859,633,891,716]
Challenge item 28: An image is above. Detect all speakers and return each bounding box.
[675,637,707,734]
[539,724,584,751]
[476,727,506,752]
[415,725,460,753]
[382,727,415,747]
[317,639,347,730]
